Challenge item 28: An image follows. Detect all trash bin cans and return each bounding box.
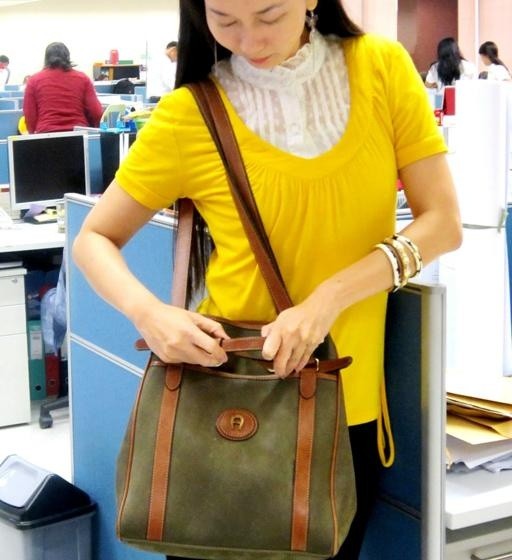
[1,453,99,558]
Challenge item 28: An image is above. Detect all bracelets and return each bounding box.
[389,232,422,279]
[381,237,410,287]
[374,243,401,295]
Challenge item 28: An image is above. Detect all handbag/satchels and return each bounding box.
[114,298,360,560]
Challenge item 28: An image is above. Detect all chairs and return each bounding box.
[38,242,69,427]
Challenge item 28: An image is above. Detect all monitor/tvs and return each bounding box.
[6,131,90,215]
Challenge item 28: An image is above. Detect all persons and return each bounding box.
[95,73,109,81]
[23,42,104,134]
[113,79,136,95]
[0,56,11,86]
[73,1,462,560]
[479,41,512,80]
[162,41,179,92]
[425,37,479,90]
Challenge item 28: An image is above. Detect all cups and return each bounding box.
[56,200,66,232]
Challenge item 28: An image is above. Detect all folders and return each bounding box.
[40,282,60,401]
[26,288,47,400]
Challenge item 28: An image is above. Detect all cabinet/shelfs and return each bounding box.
[1,269,31,427]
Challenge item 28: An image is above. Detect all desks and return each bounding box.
[0,222,66,253]
[444,468,512,531]
